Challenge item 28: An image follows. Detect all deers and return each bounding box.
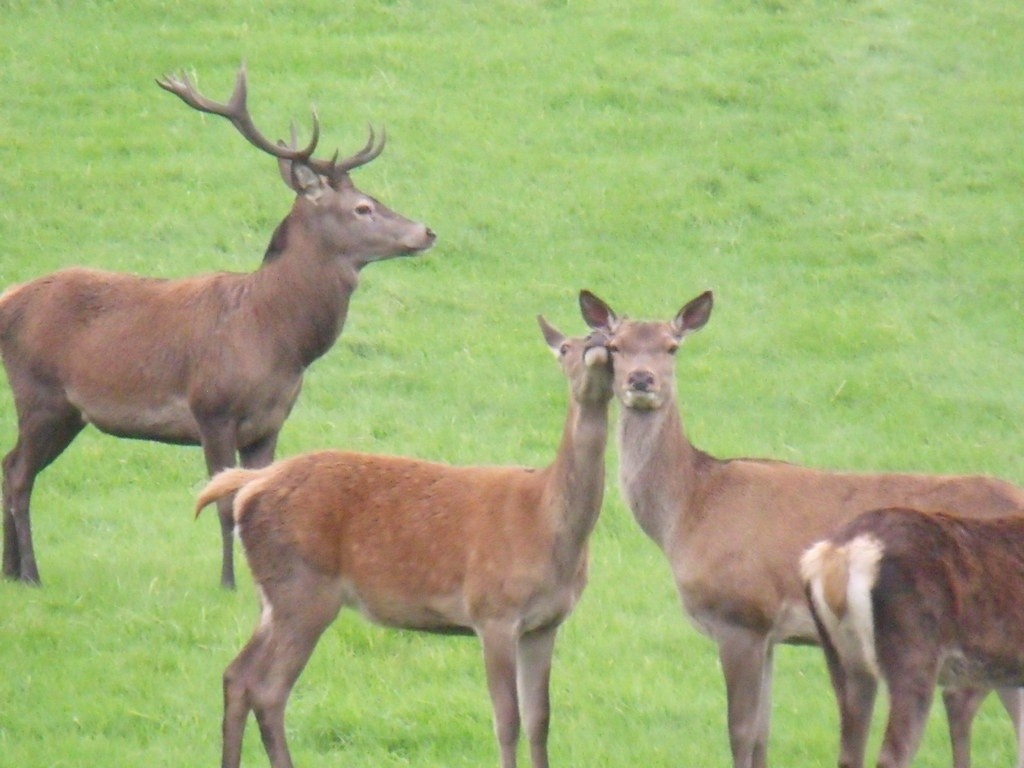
[0,59,435,584]
[579,288,1024,768]
[191,310,615,768]
